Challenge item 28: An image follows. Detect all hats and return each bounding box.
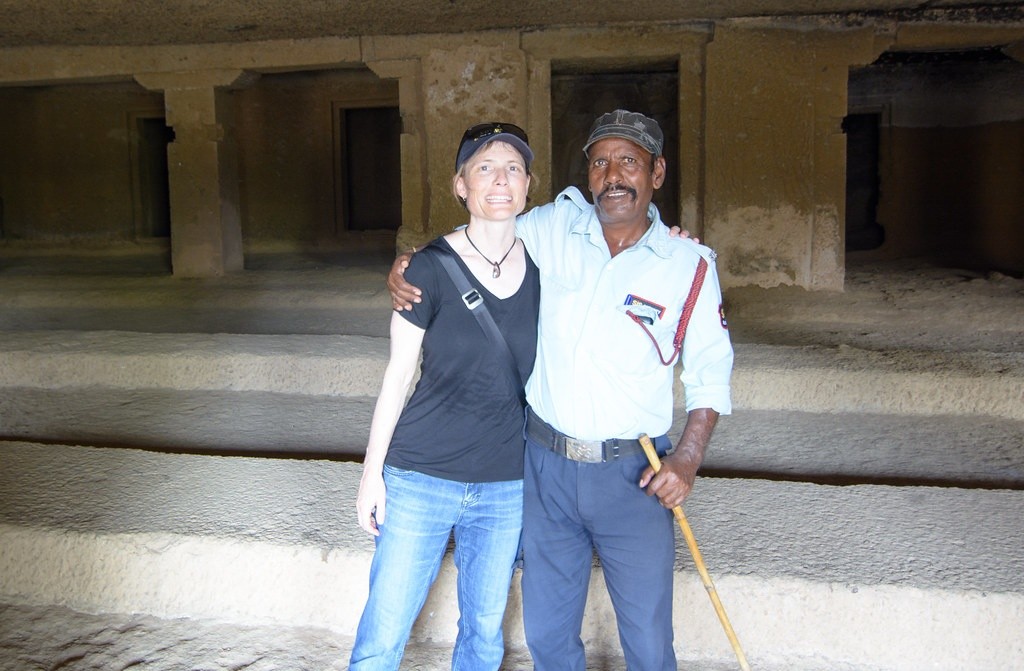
[456,123,535,174]
[582,109,663,160]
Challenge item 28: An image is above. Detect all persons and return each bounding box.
[384,107,735,671]
[348,123,543,671]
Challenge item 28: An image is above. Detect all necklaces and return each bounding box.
[464,223,518,277]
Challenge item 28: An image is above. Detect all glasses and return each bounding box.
[456,122,529,166]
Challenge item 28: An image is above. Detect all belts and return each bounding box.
[527,414,656,464]
